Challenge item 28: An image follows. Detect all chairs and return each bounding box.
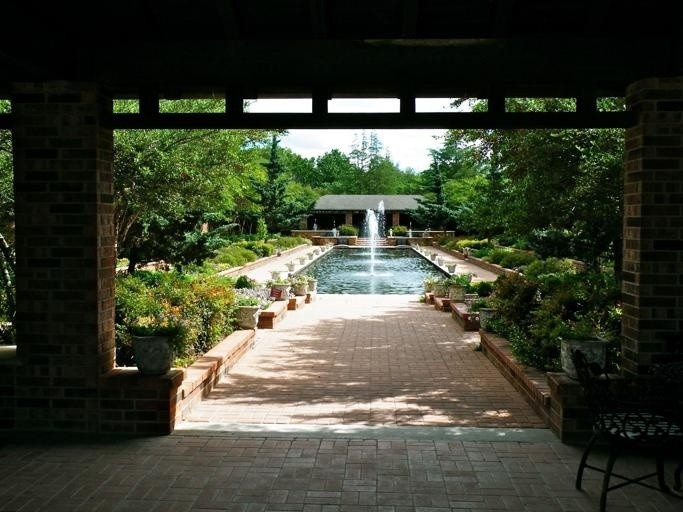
[572,348,683,512]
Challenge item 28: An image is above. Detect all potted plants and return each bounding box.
[128,274,316,376]
[423,273,623,381]
